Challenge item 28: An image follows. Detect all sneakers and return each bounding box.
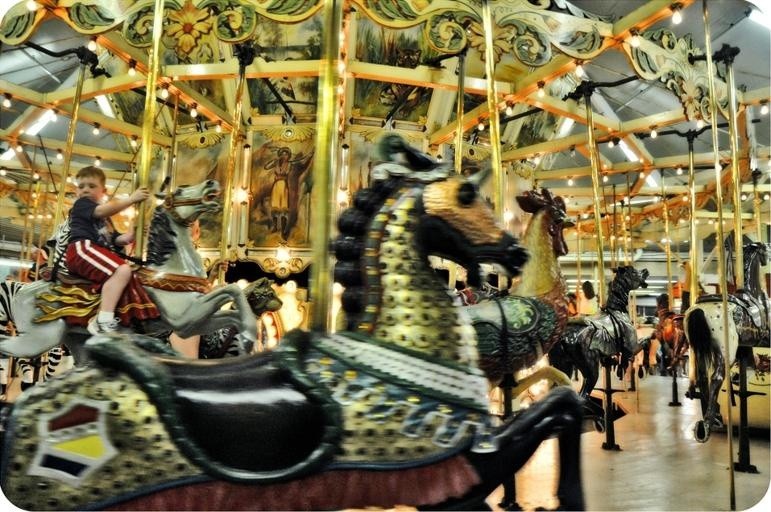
[86,313,118,336]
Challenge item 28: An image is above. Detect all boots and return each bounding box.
[280,217,289,242]
[271,217,279,234]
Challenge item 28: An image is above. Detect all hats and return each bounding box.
[276,146,293,158]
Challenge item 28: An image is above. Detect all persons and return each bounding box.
[567,282,599,313]
[264,145,314,241]
[644,317,654,324]
[66,165,149,336]
[26,247,48,281]
